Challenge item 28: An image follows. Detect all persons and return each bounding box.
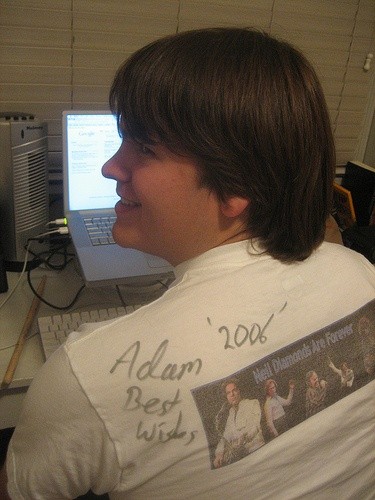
[0,26,375,500]
[325,355,356,398]
[213,376,267,467]
[303,370,328,420]
[262,378,296,440]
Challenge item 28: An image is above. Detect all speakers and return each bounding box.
[0,111,51,263]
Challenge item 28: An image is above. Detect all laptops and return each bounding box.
[60,109,177,288]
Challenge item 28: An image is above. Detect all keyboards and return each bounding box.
[35,302,151,364]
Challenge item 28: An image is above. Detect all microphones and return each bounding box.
[214,400,228,421]
